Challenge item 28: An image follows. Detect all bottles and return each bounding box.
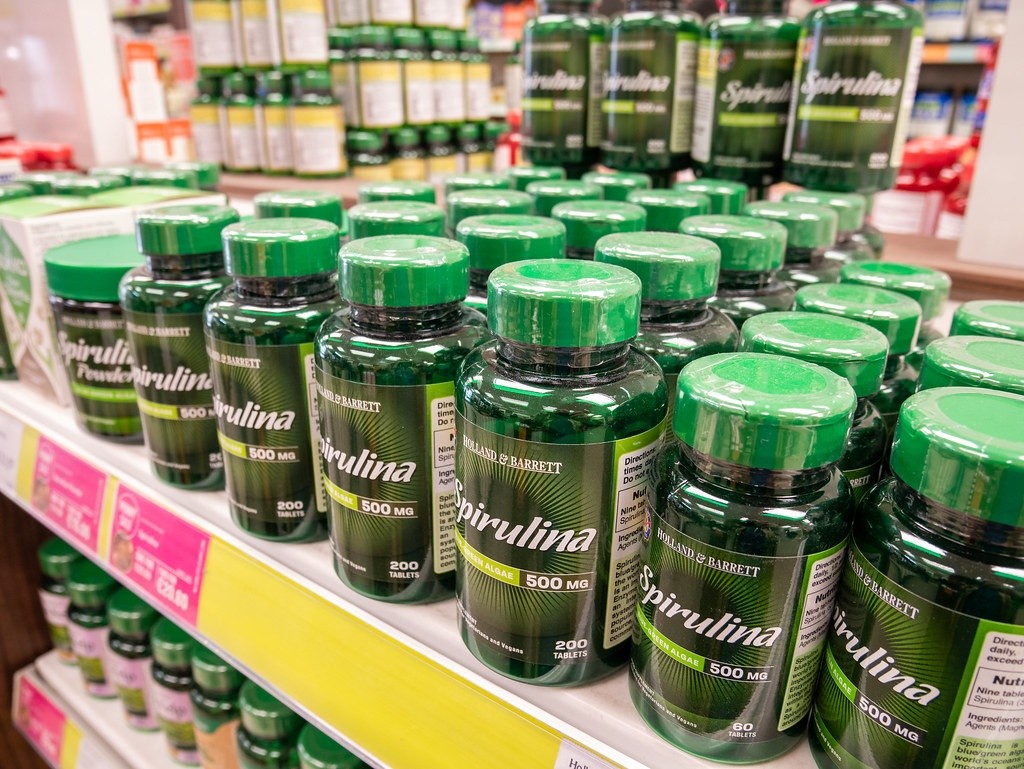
[2,0,1024,769]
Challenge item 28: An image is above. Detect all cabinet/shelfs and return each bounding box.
[0,233,1023,769]
